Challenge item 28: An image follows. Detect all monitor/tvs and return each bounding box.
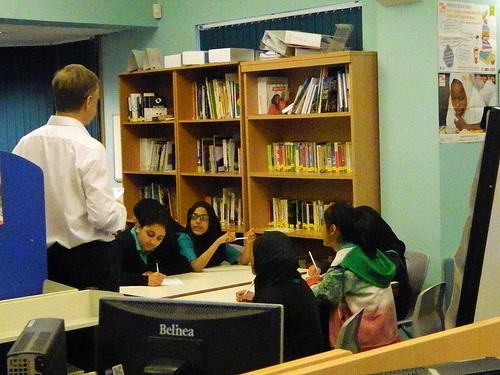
[98,298,283,375]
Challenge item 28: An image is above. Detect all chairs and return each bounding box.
[413,279,450,339]
[332,307,366,354]
[440,256,456,313]
[396,250,432,339]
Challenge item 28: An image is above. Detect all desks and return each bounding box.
[119,262,328,304]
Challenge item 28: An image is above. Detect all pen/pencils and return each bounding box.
[155,263,159,273]
[245,281,254,294]
[235,236,249,240]
[309,251,318,270]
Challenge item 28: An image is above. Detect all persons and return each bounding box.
[12,64,127,290]
[116,212,167,286]
[305,201,401,354]
[236,231,330,362]
[355,206,410,329]
[446,74,485,135]
[174,200,256,273]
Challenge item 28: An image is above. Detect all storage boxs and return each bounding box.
[182,51,209,65]
[208,48,255,62]
[164,53,187,69]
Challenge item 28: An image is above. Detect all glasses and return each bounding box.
[191,213,208,221]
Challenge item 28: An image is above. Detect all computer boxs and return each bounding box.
[5,318,66,375]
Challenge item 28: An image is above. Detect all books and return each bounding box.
[125,66,352,234]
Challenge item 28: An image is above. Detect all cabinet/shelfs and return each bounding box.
[239,50,381,267]
[173,58,250,248]
[116,67,180,230]
